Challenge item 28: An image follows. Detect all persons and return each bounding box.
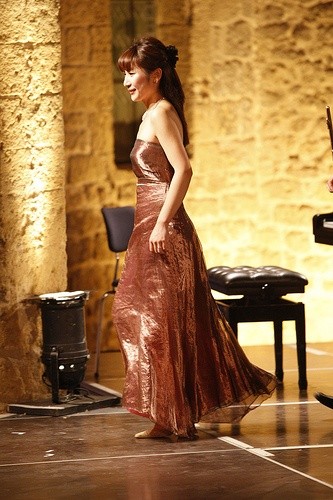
[327,177,333,193]
[111,35,277,442]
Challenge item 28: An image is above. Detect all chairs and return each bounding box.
[94,206,134,378]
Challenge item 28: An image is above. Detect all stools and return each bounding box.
[205,266,308,390]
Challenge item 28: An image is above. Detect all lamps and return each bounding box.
[37,289,90,404]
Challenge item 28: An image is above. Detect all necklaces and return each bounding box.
[141,97,162,121]
